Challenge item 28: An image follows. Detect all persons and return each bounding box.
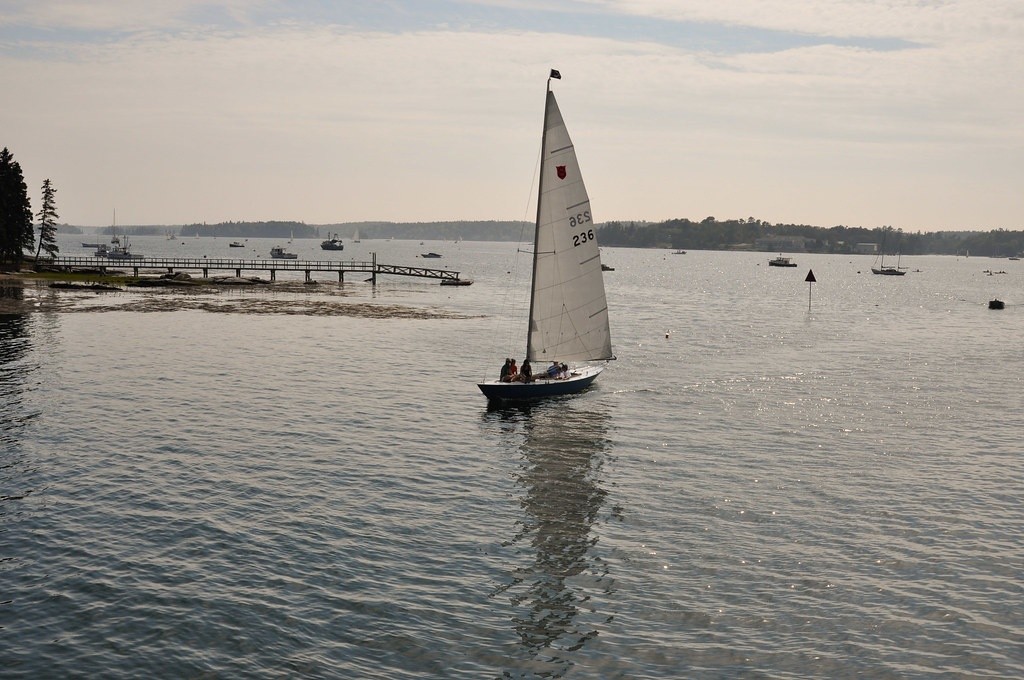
[498,354,572,384]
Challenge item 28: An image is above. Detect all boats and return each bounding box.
[320,231,344,250]
[94,244,113,256]
[988,299,1005,311]
[421,252,442,258]
[769,252,796,267]
[673,249,687,254]
[82,243,106,247]
[1008,257,1021,260]
[270,245,298,259]
[230,241,245,247]
[107,234,144,259]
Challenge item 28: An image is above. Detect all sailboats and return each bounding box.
[110,208,120,244]
[872,229,907,276]
[476,68,615,405]
